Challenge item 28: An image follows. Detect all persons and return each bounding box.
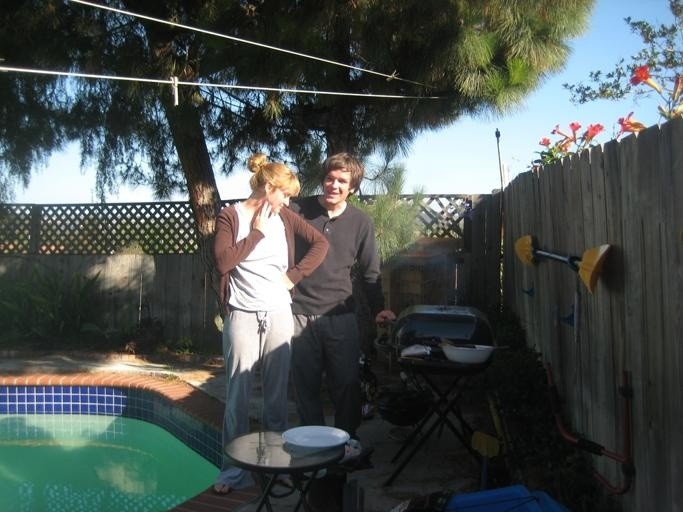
[289,151,397,480]
[212,153,331,497]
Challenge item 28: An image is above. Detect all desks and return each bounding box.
[385,347,482,485]
[224,427,345,512]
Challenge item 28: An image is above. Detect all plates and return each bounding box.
[281,425,351,449]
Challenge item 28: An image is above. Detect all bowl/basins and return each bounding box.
[440,343,496,365]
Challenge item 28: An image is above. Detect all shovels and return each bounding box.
[514,233,612,297]
[487,394,524,481]
